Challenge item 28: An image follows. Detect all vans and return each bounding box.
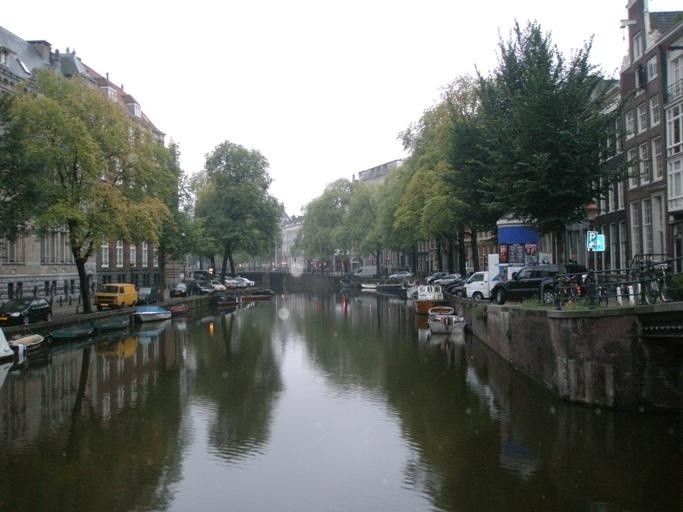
[95,337,139,360]
[93,282,138,310]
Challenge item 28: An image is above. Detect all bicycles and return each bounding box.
[598,281,608,306]
[644,264,675,303]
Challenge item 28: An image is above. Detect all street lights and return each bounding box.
[181,259,187,283]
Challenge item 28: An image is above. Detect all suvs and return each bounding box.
[491,264,594,304]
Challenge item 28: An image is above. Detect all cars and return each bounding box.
[0,297,52,326]
[138,286,164,305]
[389,270,415,279]
[170,270,255,297]
[424,272,466,297]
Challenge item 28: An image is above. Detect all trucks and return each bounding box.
[465,266,524,300]
[355,265,376,276]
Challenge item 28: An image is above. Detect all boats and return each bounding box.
[340,278,467,333]
[0,314,130,362]
[213,289,275,301]
[170,305,190,314]
[133,306,171,322]
